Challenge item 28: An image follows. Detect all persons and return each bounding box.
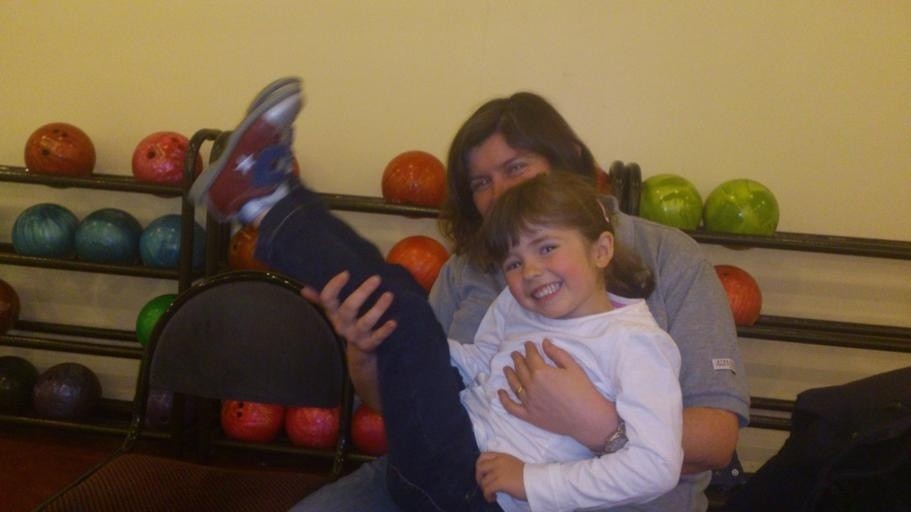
[186,78,686,512]
[285,89,751,512]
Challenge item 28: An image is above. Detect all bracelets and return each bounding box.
[595,421,629,455]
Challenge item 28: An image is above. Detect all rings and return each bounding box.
[516,386,524,394]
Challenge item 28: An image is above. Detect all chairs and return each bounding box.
[34,269,354,512]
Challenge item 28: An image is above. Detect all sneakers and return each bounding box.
[185,75,303,222]
